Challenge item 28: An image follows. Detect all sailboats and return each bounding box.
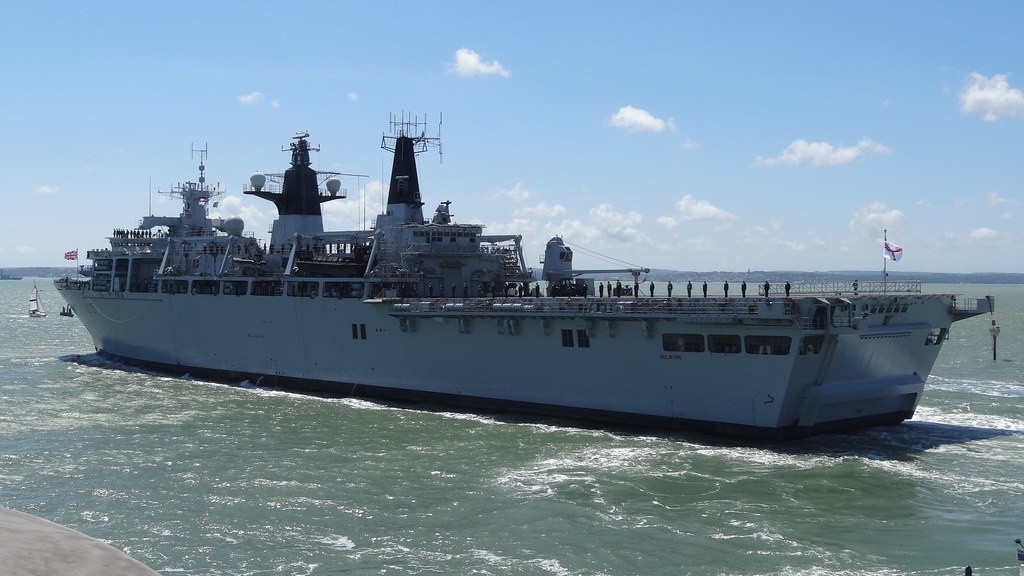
[28,278,48,318]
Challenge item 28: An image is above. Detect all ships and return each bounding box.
[52,108,1000,444]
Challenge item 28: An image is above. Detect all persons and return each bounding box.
[429,282,433,297]
[203,241,294,255]
[492,283,496,298]
[668,281,672,297]
[785,280,791,296]
[764,281,770,297]
[852,279,859,295]
[137,278,182,294]
[519,283,522,298]
[504,284,509,299]
[297,241,372,259]
[687,281,692,297]
[741,281,747,297]
[113,228,152,238]
[617,280,621,298]
[464,280,468,298]
[607,281,611,298]
[536,283,539,298]
[337,283,420,299]
[438,280,444,298]
[583,281,587,298]
[599,282,603,298]
[452,280,456,298]
[552,282,556,298]
[291,282,304,297]
[724,281,729,298]
[703,281,707,297]
[478,285,482,298]
[634,281,639,297]
[650,282,654,297]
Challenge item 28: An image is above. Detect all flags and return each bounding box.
[64,250,77,260]
[883,241,903,261]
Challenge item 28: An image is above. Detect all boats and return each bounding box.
[279,240,368,278]
[0,268,24,281]
[233,256,267,270]
